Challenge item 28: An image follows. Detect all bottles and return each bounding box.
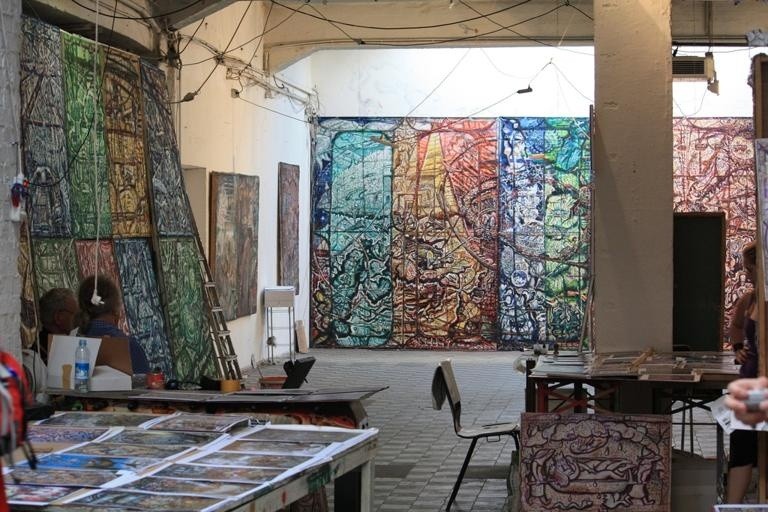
[73,339,91,394]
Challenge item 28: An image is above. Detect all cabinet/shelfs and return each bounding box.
[264,286,296,365]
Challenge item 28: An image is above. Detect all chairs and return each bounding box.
[439,360,521,511]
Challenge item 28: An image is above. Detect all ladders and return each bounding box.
[186,193,246,391]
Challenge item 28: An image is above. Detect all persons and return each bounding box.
[722,241,758,511]
[73,275,151,377]
[30,287,80,366]
[722,374,767,428]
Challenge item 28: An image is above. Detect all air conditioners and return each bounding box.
[672,56,715,82]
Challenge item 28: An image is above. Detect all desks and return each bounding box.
[528,351,742,456]
[2,384,390,511]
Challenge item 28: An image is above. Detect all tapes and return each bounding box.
[220,380,242,393]
